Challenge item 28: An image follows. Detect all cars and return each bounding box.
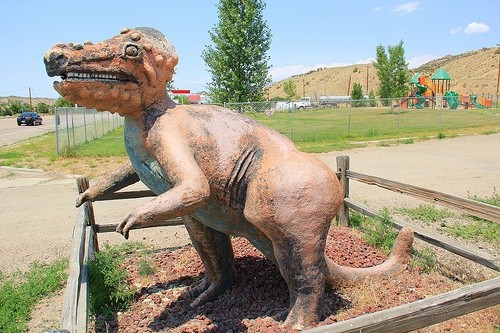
[17,112,42,126]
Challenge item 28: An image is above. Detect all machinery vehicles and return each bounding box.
[293,96,371,111]
